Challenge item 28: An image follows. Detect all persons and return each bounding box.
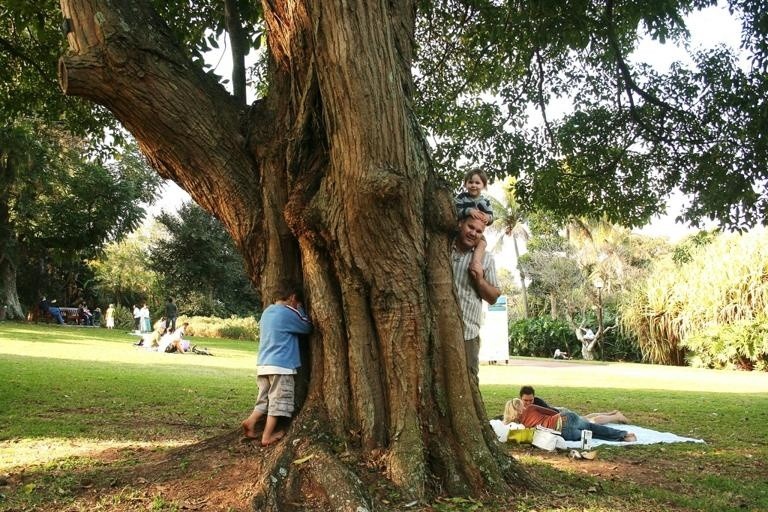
[240,279,314,446]
[553,346,573,360]
[502,397,636,443]
[105,303,115,328]
[141,304,152,332]
[519,386,632,425]
[132,303,140,324]
[449,211,503,358]
[453,168,494,278]
[39,295,64,324]
[139,319,189,352]
[75,301,98,327]
[165,298,176,327]
[578,325,595,347]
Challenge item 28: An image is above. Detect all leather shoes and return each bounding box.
[570,450,597,460]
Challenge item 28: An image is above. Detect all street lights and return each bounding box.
[594,276,606,359]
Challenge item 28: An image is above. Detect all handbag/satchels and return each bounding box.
[507,428,535,444]
[532,425,562,451]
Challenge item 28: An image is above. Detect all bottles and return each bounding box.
[581,430,593,451]
